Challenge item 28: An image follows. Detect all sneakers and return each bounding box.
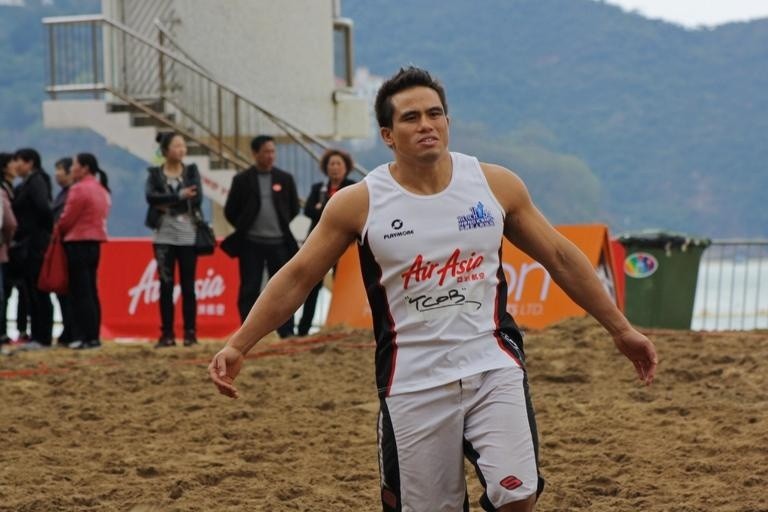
[154,337,175,348]
[184,336,197,346]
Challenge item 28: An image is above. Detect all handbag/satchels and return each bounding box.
[196,220,217,255]
[37,239,69,295]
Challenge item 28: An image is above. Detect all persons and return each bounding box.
[297,148,358,334]
[219,135,301,339]
[0,147,111,348]
[143,131,204,348]
[207,65,658,512]
